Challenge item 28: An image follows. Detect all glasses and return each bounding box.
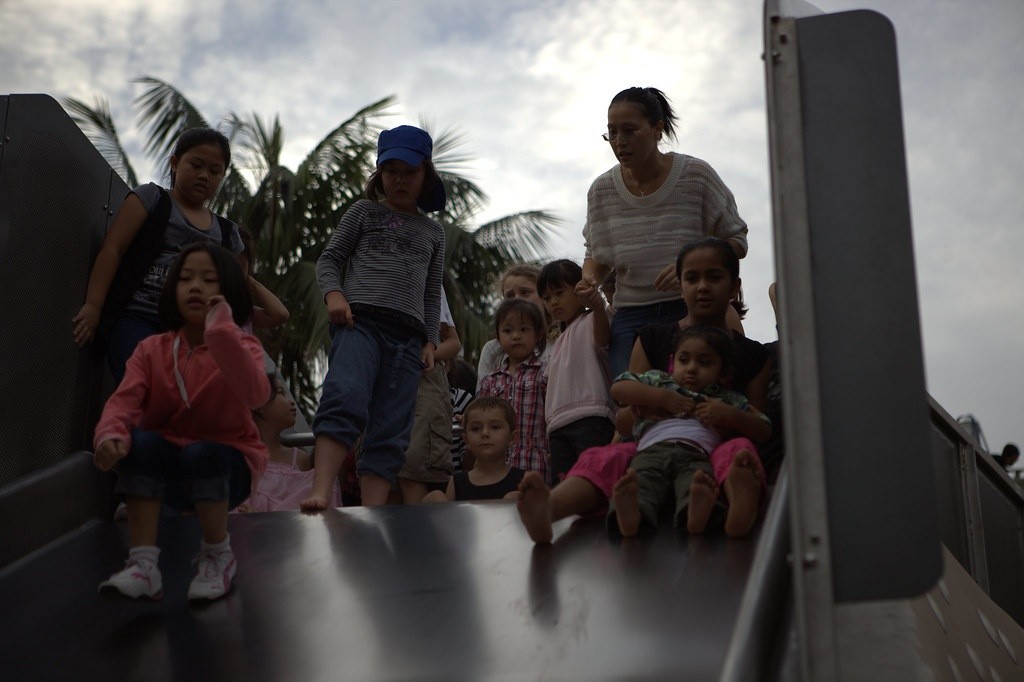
[603,121,655,142]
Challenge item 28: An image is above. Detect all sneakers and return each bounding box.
[97,559,165,603]
[188,546,238,601]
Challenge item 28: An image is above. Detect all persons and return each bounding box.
[92,239,274,606]
[580,85,750,385]
[990,444,1019,474]
[299,124,447,514]
[225,235,787,544]
[70,126,252,522]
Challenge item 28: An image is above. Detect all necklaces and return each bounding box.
[629,168,653,197]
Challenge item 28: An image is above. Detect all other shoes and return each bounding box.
[115,502,129,526]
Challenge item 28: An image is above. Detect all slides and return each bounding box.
[0,446,796,682]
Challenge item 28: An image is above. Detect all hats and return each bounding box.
[376,124,446,213]
